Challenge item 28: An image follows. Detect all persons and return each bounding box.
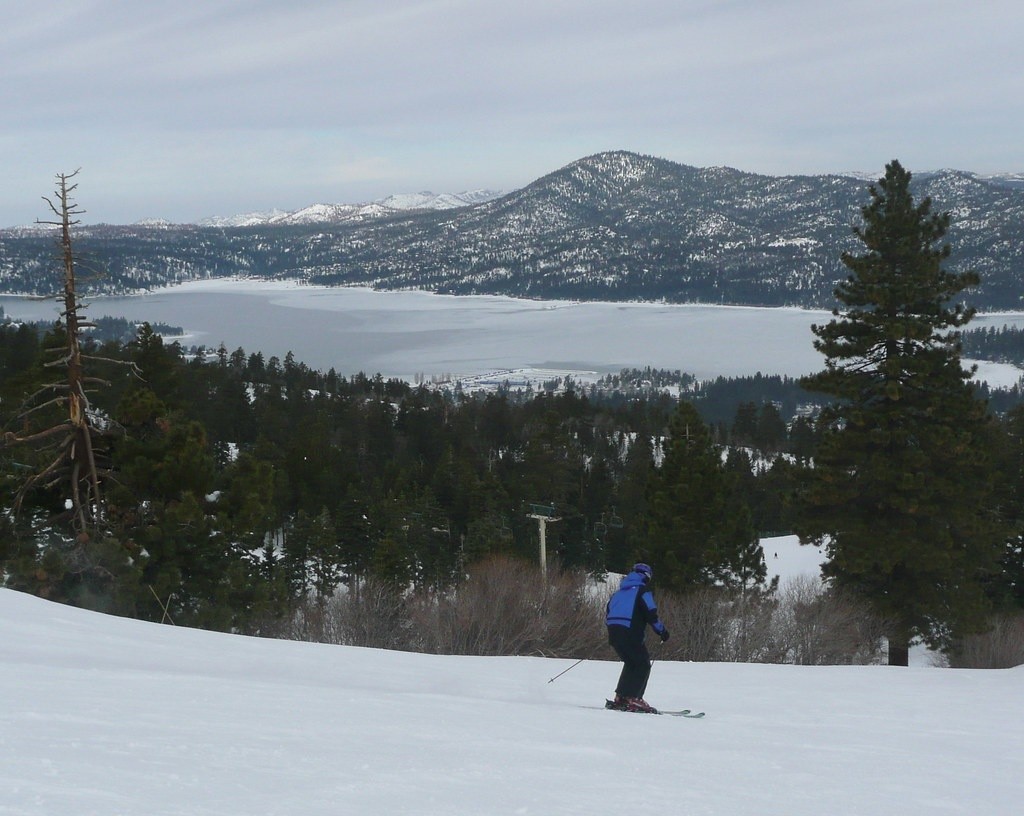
[606,563,669,714]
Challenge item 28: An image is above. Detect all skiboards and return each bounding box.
[604,698,705,718]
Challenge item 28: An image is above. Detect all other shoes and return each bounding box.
[614,694,650,708]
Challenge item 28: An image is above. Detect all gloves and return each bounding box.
[660,631,670,643]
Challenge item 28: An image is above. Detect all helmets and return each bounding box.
[633,563,652,580]
